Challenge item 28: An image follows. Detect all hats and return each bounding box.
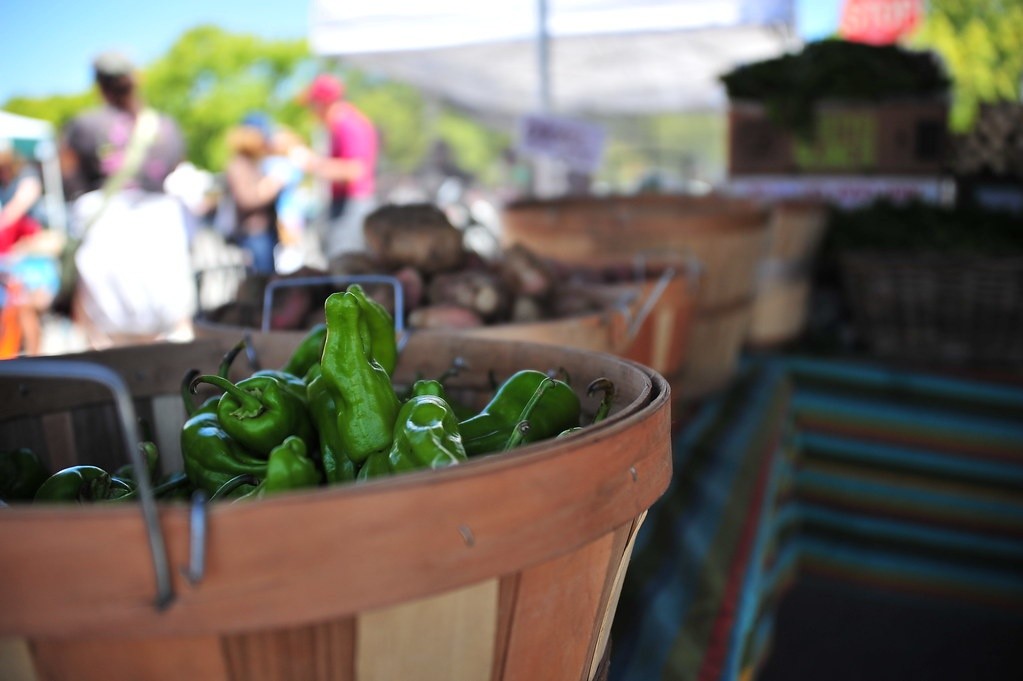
[300,74,341,104]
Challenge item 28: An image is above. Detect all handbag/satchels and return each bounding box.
[65,190,106,238]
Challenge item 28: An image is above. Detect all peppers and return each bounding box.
[0,282,615,504]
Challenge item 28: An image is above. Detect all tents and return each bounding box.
[307,1,805,127]
[0,110,64,230]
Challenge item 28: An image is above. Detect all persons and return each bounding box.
[0,54,380,360]
[410,142,467,175]
[501,149,535,207]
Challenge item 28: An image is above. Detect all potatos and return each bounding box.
[219,205,597,328]
